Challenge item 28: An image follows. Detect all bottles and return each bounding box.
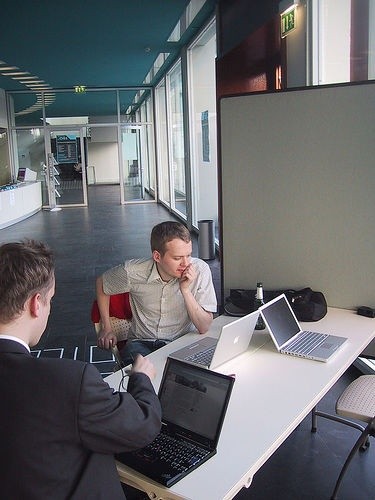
[253,282,267,330]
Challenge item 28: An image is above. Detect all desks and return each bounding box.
[103,306,375,500]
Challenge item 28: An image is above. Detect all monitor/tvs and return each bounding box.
[18,167,37,182]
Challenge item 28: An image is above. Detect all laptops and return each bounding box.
[258,293,350,362]
[169,308,260,370]
[115,357,235,488]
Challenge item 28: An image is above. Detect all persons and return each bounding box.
[95,221,217,366]
[0,238,162,500]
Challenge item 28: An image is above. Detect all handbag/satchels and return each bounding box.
[223,283,327,322]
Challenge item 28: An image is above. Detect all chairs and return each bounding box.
[91,293,132,367]
[331,375,375,500]
